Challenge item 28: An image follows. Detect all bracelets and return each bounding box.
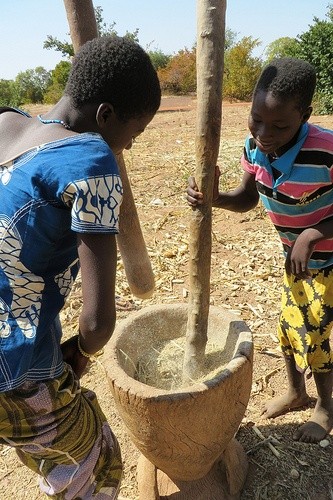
[77,334,105,360]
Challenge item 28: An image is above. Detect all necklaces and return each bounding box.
[36,112,72,130]
[267,151,284,161]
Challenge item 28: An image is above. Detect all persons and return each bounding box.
[185,58,333,443]
[0,34,163,500]
